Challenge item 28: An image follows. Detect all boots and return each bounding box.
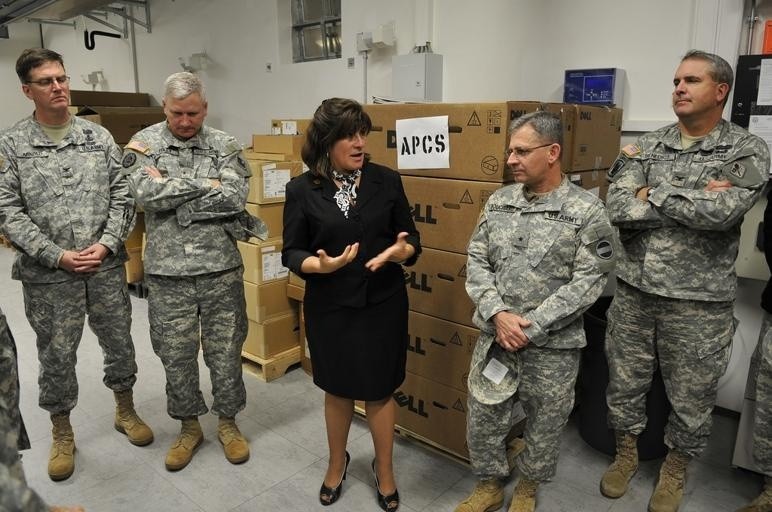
[113,389,153,446]
[164,416,203,470]
[47,413,75,481]
[455,477,504,511]
[217,414,249,463]
[507,476,539,512]
[599,430,640,498]
[647,448,693,511]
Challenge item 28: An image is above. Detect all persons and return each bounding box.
[0,308,87,512]
[0,47,154,482]
[600,49,771,512]
[453,111,618,512]
[121,72,269,472]
[281,98,423,512]
[736,188,772,512]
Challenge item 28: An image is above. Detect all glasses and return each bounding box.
[505,143,553,156]
[24,76,70,85]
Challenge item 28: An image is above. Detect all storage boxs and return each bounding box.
[239,117,313,362]
[69,89,165,281]
[354,99,624,476]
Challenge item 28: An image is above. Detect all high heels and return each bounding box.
[318,449,350,505]
[371,457,398,511]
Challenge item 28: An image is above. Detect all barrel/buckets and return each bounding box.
[577,296,674,462]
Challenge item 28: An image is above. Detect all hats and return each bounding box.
[221,208,268,244]
[466,333,522,405]
